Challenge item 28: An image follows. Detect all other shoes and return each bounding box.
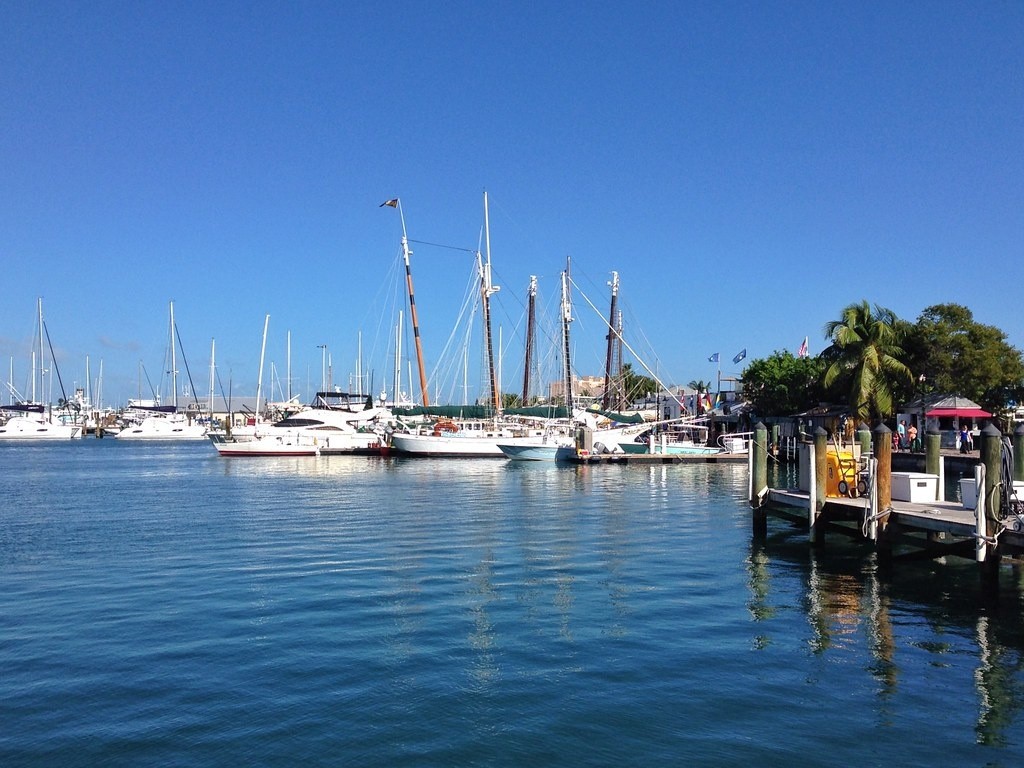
[968,452,972,454]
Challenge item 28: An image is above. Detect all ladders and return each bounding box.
[479,293,496,428]
[609,308,622,376]
[559,304,576,416]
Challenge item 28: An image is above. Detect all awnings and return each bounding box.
[926,408,991,418]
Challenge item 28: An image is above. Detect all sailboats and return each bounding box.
[0,188,753,455]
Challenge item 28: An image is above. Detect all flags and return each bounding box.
[733,348,746,365]
[680,390,720,410]
[381,198,399,208]
[799,339,807,356]
[708,352,719,361]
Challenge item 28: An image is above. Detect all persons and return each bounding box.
[907,423,917,453]
[897,420,906,452]
[893,431,898,451]
[959,425,972,454]
